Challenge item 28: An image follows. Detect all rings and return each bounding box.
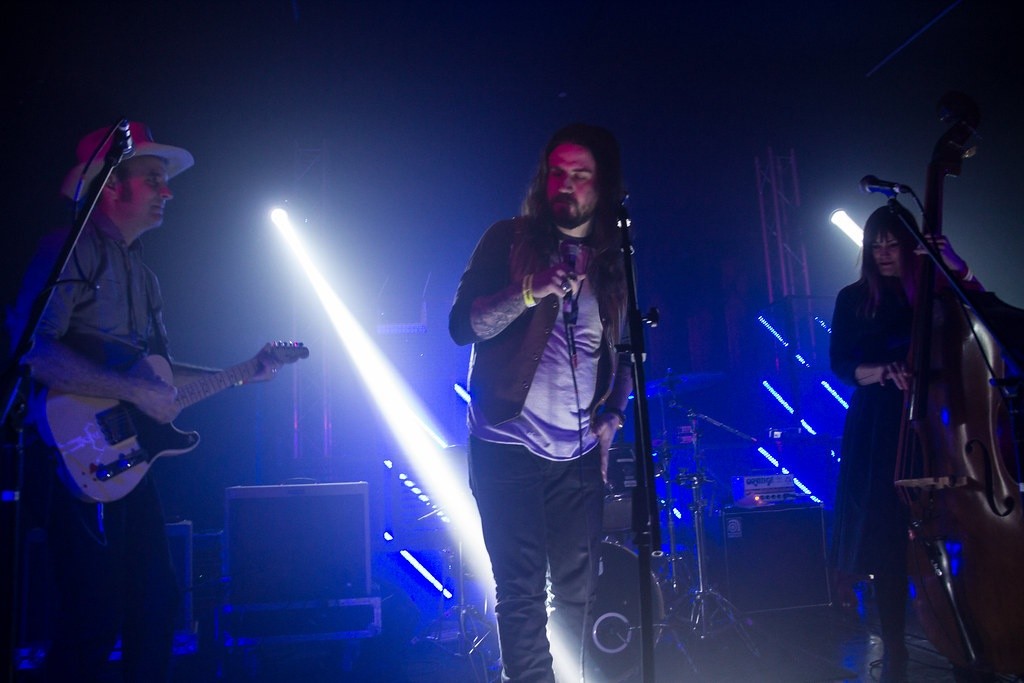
[271,368,278,374]
[560,281,571,292]
[566,271,577,281]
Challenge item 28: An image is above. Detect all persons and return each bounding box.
[829,204,987,683]
[448,121,641,683]
[0,122,285,683]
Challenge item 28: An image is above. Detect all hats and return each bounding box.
[62,126,194,204]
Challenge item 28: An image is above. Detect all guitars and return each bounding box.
[32,341,311,502]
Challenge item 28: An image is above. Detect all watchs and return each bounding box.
[602,404,627,431]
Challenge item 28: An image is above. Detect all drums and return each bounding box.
[601,440,645,531]
[593,535,667,679]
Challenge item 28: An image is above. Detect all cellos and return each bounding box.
[881,116,1024,681]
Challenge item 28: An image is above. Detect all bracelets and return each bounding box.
[522,273,542,308]
[961,267,974,282]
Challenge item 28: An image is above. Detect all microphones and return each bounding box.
[559,238,580,325]
[115,116,136,159]
[860,174,912,196]
